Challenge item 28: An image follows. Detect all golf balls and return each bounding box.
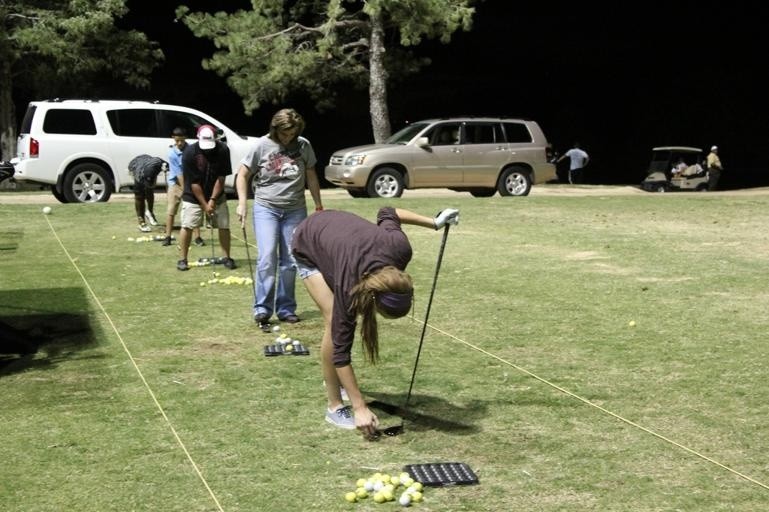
[628,321,636,326]
[188,258,211,268]
[112,236,115,239]
[273,326,280,332]
[201,272,252,286]
[276,334,300,351]
[128,234,175,244]
[43,206,51,214]
[345,471,424,507]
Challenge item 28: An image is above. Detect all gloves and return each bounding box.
[434,207,461,229]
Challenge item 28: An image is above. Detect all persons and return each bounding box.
[162,126,204,247]
[668,145,721,189]
[555,141,591,185]
[290,206,460,435]
[178,124,235,270]
[0,159,15,183]
[237,108,325,326]
[128,154,169,232]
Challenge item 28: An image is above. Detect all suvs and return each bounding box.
[9,95,262,204]
[323,114,560,200]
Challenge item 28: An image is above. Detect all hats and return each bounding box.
[143,167,157,189]
[196,124,218,149]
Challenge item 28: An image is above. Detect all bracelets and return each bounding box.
[210,197,216,202]
[316,207,323,211]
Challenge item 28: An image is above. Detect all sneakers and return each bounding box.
[162,236,172,248]
[280,314,300,324]
[193,237,205,247]
[324,378,350,400]
[138,220,152,234]
[222,257,237,270]
[325,404,359,430]
[145,211,159,226]
[177,259,190,271]
[256,313,271,325]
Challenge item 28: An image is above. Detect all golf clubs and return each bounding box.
[384,223,450,436]
[210,214,216,272]
[239,219,271,328]
